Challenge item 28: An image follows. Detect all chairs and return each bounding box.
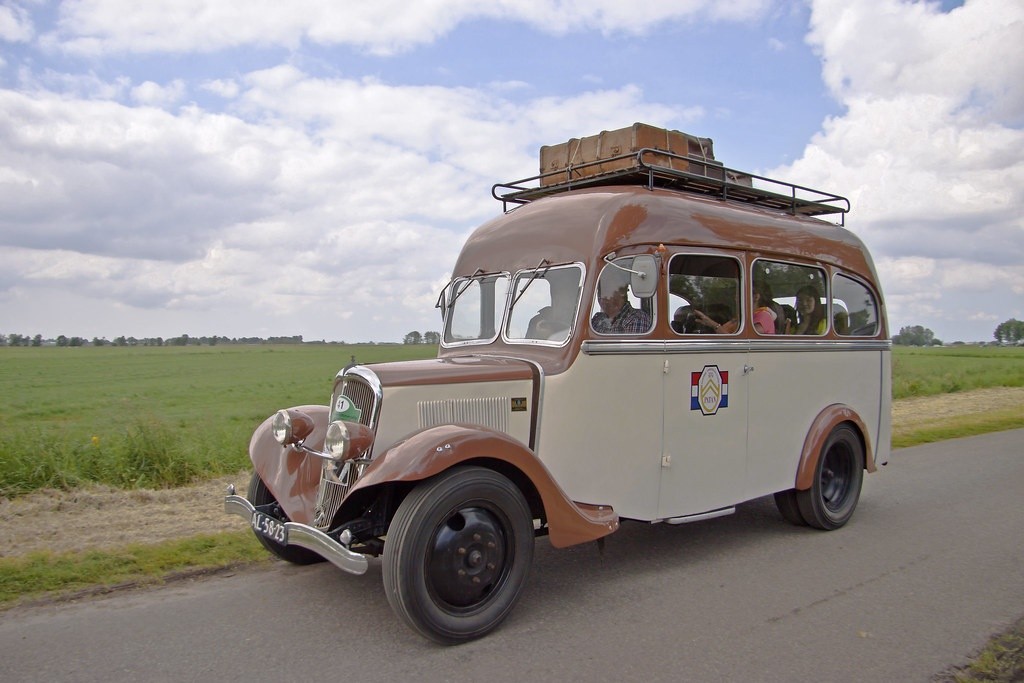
[695,303,847,334]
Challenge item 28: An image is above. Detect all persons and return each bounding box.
[524,267,826,339]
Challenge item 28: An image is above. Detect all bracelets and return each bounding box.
[714,324,720,330]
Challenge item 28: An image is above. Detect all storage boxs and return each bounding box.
[540,123,752,187]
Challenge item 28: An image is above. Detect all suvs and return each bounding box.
[223,145,893,646]
